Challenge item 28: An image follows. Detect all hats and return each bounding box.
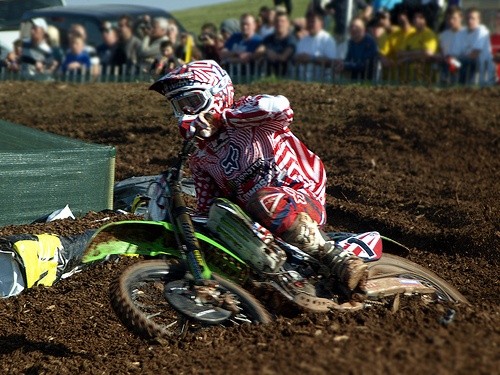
[32,18,47,34]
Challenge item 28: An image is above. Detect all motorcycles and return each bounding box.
[66,113,475,347]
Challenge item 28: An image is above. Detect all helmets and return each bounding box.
[148,59,235,140]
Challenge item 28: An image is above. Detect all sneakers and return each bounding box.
[320,242,368,293]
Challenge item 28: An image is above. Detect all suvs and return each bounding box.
[13,3,186,64]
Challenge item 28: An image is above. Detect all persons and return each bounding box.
[147,59,368,291]
[0,6,494,77]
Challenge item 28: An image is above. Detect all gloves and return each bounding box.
[185,104,226,141]
